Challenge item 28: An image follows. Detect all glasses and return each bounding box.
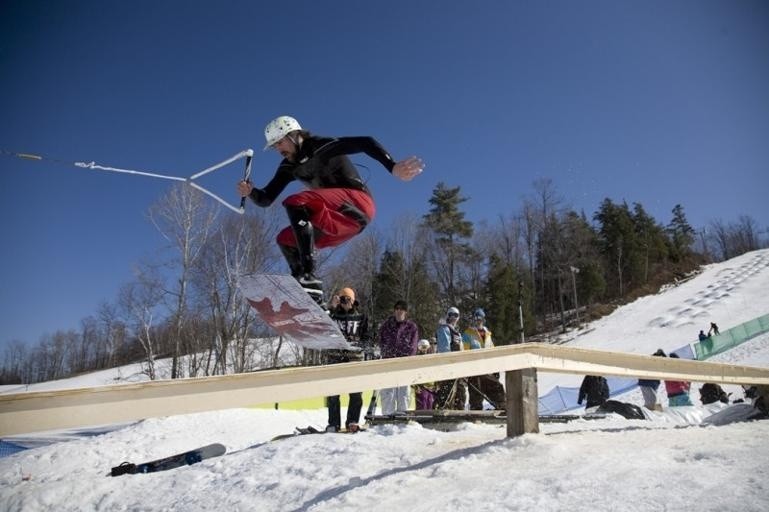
[448,312,459,318]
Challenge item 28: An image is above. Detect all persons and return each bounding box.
[699,328,708,354]
[321,287,369,432]
[636,350,668,408]
[415,335,433,408]
[434,305,464,409]
[708,321,721,335]
[706,333,714,351]
[576,374,610,409]
[660,353,696,405]
[461,308,507,410]
[378,301,420,415]
[237,113,426,317]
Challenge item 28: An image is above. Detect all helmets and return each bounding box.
[262,116,305,152]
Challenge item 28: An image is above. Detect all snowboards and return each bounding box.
[239,274,352,350]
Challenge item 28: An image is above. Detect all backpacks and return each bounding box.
[699,382,730,405]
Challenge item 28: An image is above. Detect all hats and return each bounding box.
[393,301,408,311]
[447,307,460,320]
[338,287,356,303]
[474,308,486,318]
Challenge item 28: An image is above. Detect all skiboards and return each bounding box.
[111,444,226,475]
[295,425,365,435]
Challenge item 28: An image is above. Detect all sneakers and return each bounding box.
[326,420,362,432]
[295,272,323,305]
